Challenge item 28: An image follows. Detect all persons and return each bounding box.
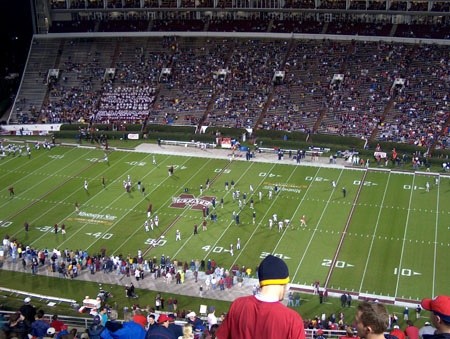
[214,255,309,339]
[0,292,227,338]
[288,301,362,339]
[388,303,423,339]
[353,301,390,339]
[3,19,449,300]
[420,295,450,339]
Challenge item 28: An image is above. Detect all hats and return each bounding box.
[394,325,399,329]
[258,255,289,287]
[47,327,55,334]
[94,316,100,324]
[24,297,31,304]
[158,314,172,323]
[133,314,146,327]
[421,296,450,316]
[187,311,196,317]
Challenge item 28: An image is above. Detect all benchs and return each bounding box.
[11,0,450,150]
